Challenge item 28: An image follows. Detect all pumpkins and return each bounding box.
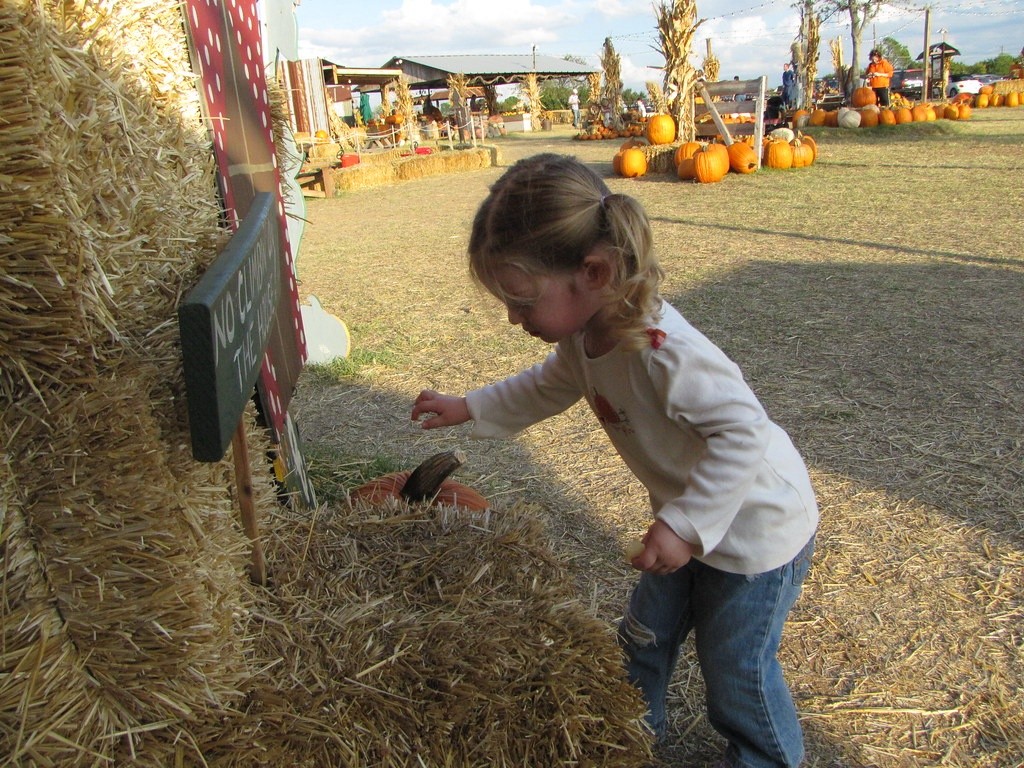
[612,109,818,184]
[575,116,649,141]
[792,80,1024,129]
[315,110,506,140]
[347,450,491,514]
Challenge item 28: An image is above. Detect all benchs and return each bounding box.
[296,162,334,199]
[364,135,392,151]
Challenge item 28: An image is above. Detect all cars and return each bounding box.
[932,72,1009,97]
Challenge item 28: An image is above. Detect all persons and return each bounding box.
[432,107,458,137]
[732,76,746,101]
[637,98,647,117]
[568,89,580,127]
[781,63,798,109]
[470,94,483,112]
[865,49,893,106]
[410,153,818,768]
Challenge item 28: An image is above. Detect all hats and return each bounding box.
[573,88,576,92]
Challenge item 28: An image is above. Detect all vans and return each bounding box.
[890,69,926,98]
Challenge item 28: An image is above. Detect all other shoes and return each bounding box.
[572,123,579,129]
